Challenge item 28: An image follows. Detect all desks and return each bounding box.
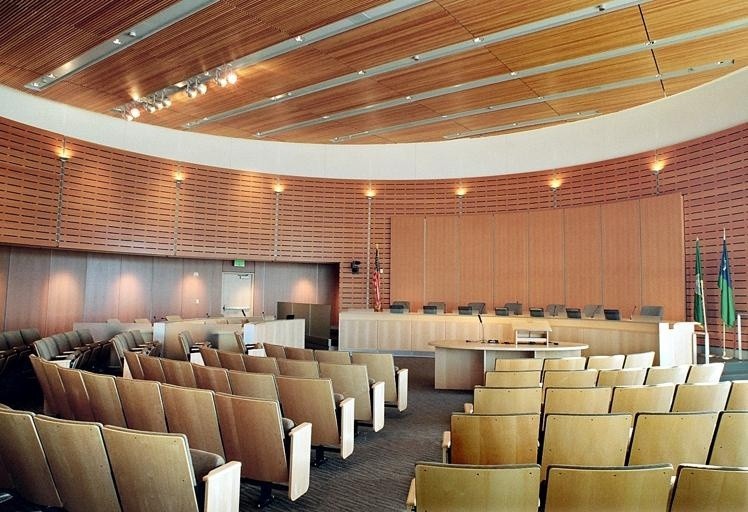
[429,340,588,392]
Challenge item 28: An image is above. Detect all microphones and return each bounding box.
[444,304,446,314]
[630,306,636,320]
[591,305,599,318]
[478,315,485,343]
[408,302,411,313]
[553,305,556,316]
[517,301,519,316]
[241,309,250,324]
[261,311,266,322]
[480,304,485,314]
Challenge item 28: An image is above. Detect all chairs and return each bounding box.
[545,304,566,316]
[405,411,747,511]
[640,305,663,320]
[0,351,356,512]
[583,304,604,318]
[393,301,410,312]
[428,302,444,313]
[482,324,517,342]
[464,352,747,413]
[0,315,409,432]
[468,303,485,314]
[504,302,522,315]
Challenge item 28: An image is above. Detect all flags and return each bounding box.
[373,249,381,313]
[694,241,704,329]
[717,240,735,327]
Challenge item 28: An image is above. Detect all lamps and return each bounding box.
[121,64,237,121]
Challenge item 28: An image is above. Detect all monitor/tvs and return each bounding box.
[423,306,436,314]
[604,310,619,320]
[530,308,543,317]
[390,305,403,313]
[458,307,472,315]
[566,309,581,318]
[495,307,508,315]
